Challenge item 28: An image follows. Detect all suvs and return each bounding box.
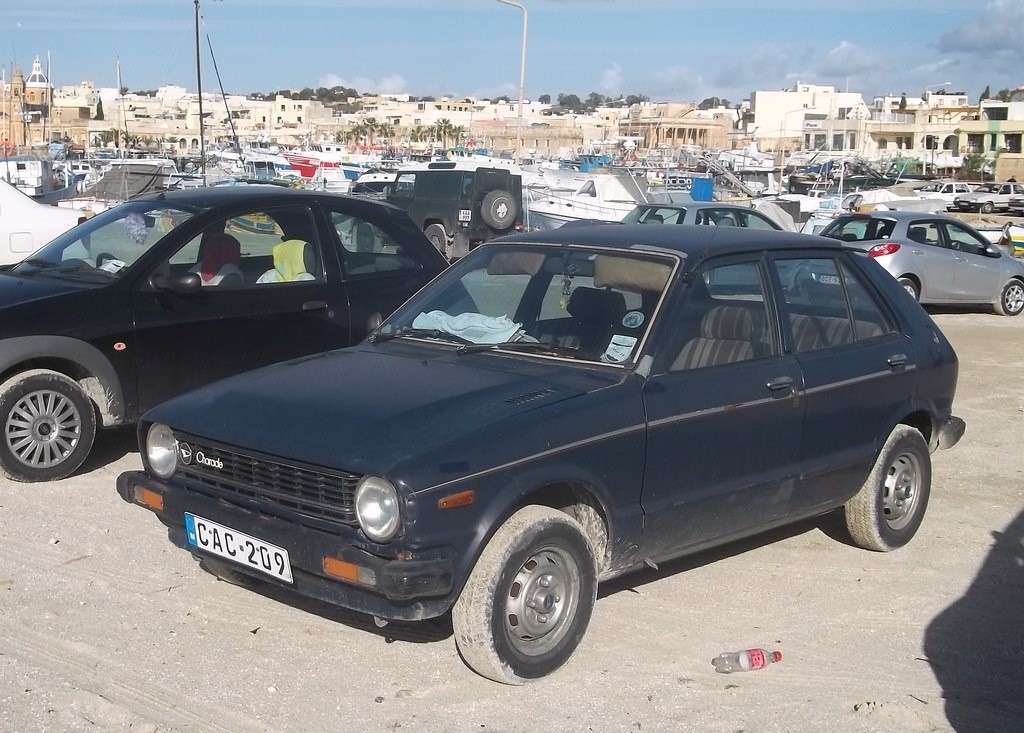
[378,159,524,262]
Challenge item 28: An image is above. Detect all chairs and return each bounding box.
[714,217,735,227]
[188,233,244,287]
[552,286,626,353]
[650,303,755,373]
[645,215,663,225]
[256,240,317,284]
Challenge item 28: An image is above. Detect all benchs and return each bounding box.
[788,315,883,351]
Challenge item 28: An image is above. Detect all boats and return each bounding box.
[210,149,375,179]
[527,173,730,230]
[570,137,903,214]
[306,166,351,192]
[355,159,415,192]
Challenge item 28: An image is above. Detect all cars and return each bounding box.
[954,181,1024,214]
[620,204,804,294]
[1008,194,1024,216]
[0,186,481,483]
[115,222,966,685]
[800,211,1024,316]
[0,178,91,267]
[915,182,974,207]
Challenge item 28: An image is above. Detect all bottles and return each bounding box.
[714,648,782,674]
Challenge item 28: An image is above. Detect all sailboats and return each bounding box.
[0,51,78,204]
[57,0,260,217]
